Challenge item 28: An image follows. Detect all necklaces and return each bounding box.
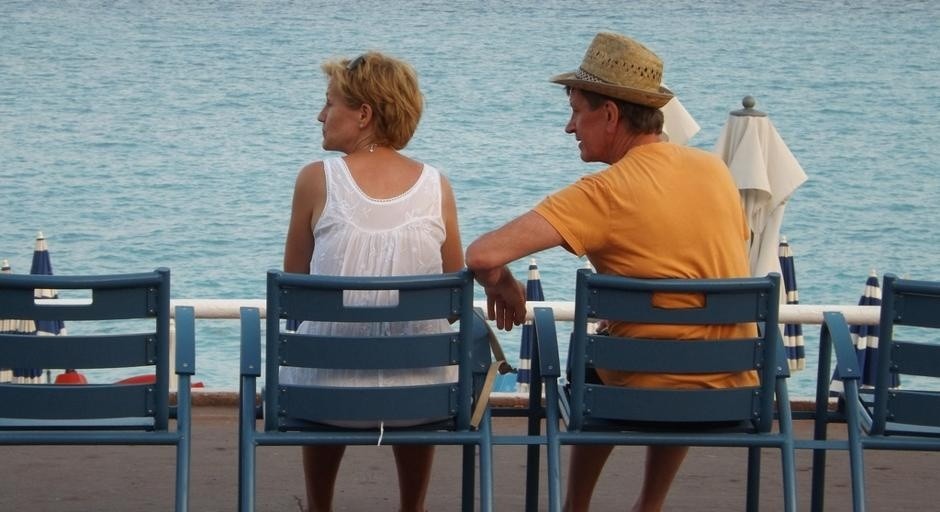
[365,142,389,151]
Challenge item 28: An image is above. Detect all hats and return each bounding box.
[550,31,674,108]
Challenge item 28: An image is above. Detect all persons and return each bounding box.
[465,32,761,512]
[284,52,466,512]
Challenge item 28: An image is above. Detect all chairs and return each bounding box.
[526,267,798,511]
[3,269,196,508]
[811,274,940,511]
[237,268,494,510]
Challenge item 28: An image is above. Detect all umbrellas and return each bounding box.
[832,269,901,388]
[778,235,805,371]
[713,95,808,342]
[0,231,65,385]
[516,258,547,395]
